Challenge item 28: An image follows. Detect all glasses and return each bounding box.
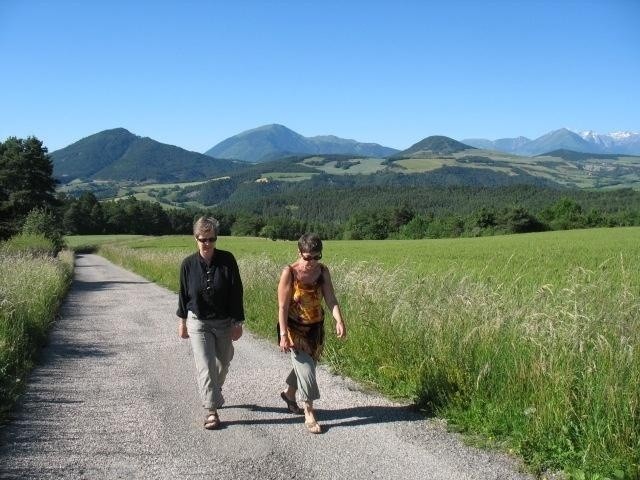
[198,237,218,243]
[301,252,322,261]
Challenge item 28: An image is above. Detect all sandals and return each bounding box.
[204,388,226,430]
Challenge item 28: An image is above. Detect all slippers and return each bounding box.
[282,391,302,414]
[304,420,323,435]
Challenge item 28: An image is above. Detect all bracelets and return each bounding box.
[233,321,243,328]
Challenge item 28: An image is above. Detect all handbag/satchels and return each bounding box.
[276,320,283,349]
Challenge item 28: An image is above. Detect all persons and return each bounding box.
[175,215,248,432]
[277,232,347,435]
